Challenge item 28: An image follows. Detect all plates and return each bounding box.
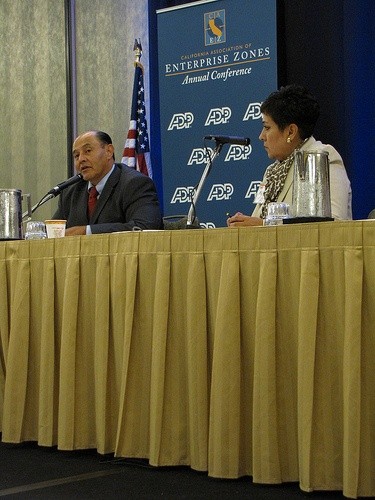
[283,217,334,224]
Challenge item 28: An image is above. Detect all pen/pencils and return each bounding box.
[226,213,232,217]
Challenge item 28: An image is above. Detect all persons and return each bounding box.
[40,131,164,238]
[227,83,353,229]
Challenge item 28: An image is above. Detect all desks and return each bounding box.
[1,218,375,498]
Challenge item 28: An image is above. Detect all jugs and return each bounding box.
[292,148,332,217]
[0,188,32,240]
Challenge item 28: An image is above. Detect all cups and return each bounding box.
[45,220,66,238]
[267,202,286,226]
[25,221,46,241]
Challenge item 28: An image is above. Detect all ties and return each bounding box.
[85,186,99,224]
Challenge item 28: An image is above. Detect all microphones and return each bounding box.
[48,173,83,194]
[204,134,250,146]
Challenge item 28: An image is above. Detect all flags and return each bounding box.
[120,61,153,179]
